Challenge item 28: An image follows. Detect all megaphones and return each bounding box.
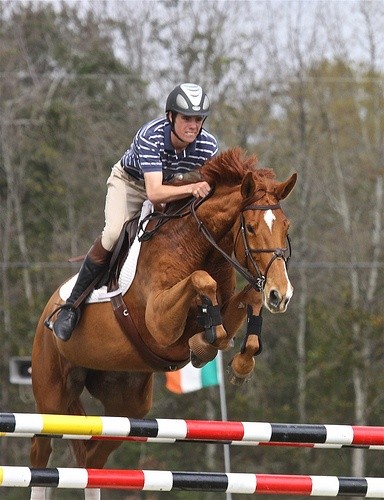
[9,356,34,384]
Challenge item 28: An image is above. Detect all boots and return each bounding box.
[52,236,113,342]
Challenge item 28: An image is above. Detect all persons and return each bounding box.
[53,82,218,343]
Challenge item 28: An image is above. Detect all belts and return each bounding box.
[120,160,139,181]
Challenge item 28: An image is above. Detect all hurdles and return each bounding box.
[1,412,384,500]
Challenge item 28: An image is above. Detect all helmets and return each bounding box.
[165,83,211,116]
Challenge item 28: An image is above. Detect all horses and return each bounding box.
[30,146,298,500]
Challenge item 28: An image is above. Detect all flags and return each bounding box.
[165,357,218,395]
